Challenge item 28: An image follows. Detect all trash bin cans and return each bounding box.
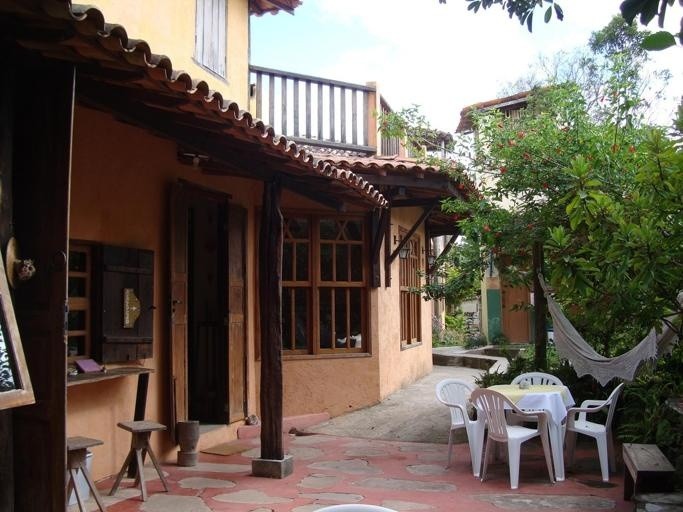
[67,450,94,505]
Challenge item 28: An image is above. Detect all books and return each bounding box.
[75,359,102,373]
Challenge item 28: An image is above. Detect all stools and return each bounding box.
[616,440,679,510]
[104,416,170,506]
[65,433,107,512]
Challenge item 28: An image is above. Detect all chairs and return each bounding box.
[435,355,629,496]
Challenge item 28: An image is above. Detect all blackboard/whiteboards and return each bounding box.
[0,264,37,411]
